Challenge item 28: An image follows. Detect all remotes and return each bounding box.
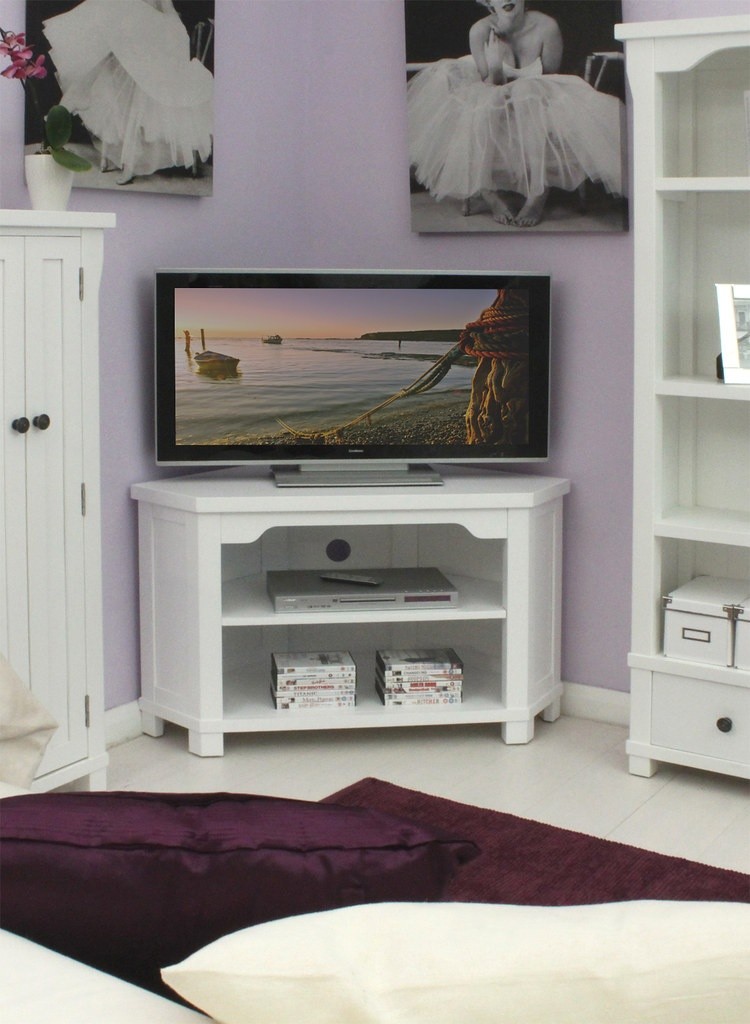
[320,571,384,586]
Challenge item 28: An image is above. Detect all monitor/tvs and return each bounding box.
[153,267,552,487]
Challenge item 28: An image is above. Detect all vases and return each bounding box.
[25,153,75,211]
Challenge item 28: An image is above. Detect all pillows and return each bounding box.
[160,898,750,1024]
[0,791,482,1016]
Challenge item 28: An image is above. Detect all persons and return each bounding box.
[404,1,624,228]
[40,1,213,189]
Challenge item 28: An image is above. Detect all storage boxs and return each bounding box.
[661,573,750,668]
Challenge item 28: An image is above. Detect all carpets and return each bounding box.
[313,776,750,908]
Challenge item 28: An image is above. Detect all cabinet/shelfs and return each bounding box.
[0,208,117,795]
[128,462,572,757]
[615,12,750,781]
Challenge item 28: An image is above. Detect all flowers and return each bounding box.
[0,27,93,173]
